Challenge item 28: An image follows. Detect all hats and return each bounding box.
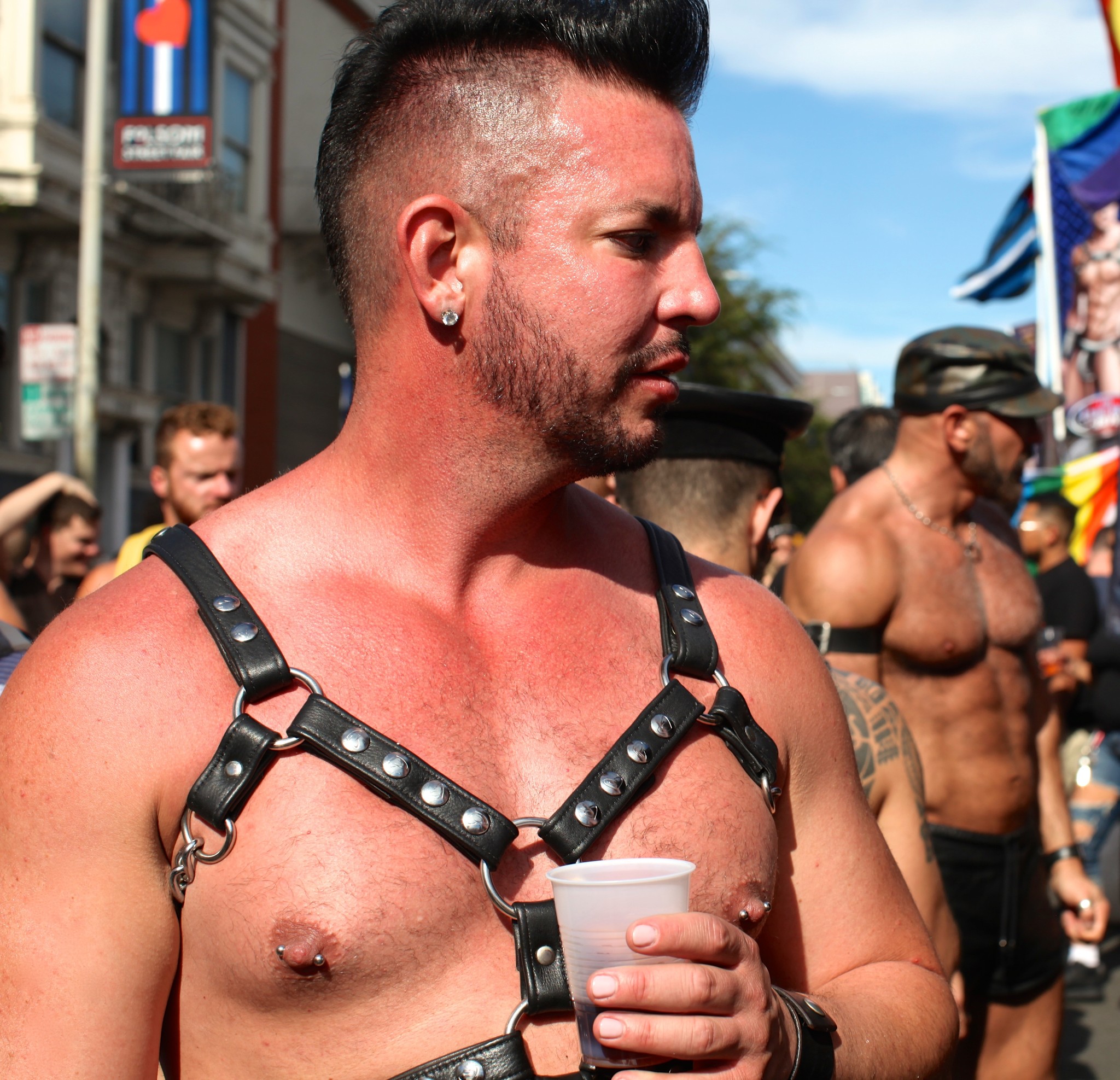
[611,381,813,470]
[894,327,1062,419]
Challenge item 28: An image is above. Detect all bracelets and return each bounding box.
[771,983,840,1080]
[1046,845,1077,871]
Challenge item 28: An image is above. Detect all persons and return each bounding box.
[2,0,1120,1080]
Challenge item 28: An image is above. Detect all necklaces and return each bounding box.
[881,460,980,559]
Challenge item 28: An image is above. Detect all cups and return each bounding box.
[1036,625,1065,678]
[544,858,695,1068]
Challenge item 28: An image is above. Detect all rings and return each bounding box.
[1077,899,1094,912]
